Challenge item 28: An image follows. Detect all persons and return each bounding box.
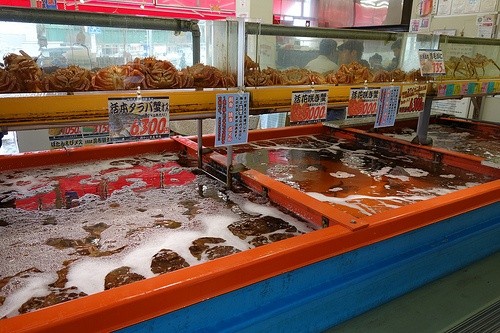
[302,40,339,75]
[337,41,369,70]
[369,39,402,71]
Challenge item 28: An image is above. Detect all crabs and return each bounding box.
[245,55,434,86]
[0,50,237,92]
[444,52,500,78]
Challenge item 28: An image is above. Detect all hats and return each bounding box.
[337,41,363,50]
